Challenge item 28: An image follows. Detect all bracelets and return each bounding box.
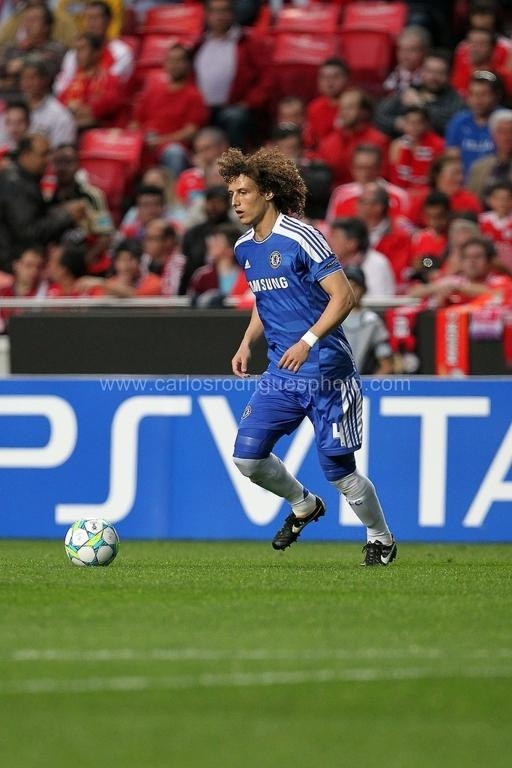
[301,330,319,348]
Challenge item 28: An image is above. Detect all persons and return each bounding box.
[0,1,511,311]
[340,264,396,376]
[214,143,396,567]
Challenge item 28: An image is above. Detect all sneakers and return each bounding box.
[272,494,325,550]
[361,533,396,566]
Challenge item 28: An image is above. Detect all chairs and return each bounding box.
[0,0,510,377]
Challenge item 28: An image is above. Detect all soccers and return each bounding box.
[64,517,120,567]
